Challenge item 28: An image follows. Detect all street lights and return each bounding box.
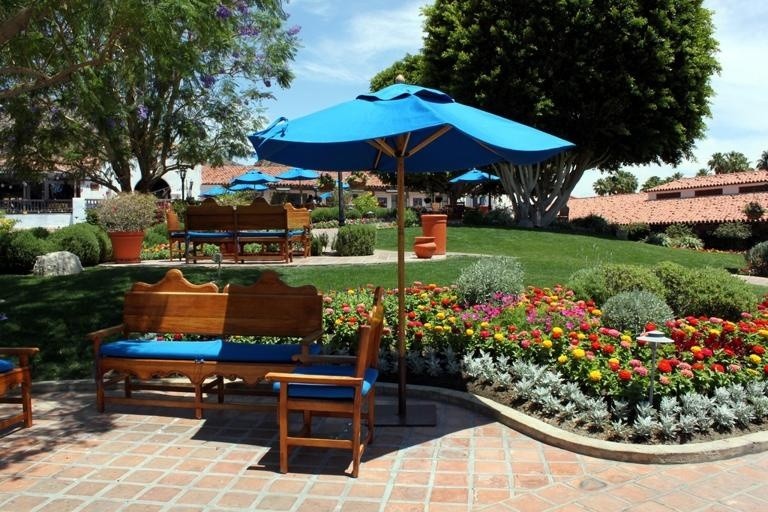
[313,185,319,199]
[179,168,188,202]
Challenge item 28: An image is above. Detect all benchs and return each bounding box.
[184,198,293,264]
[86,269,325,419]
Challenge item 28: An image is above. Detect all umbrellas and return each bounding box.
[448,167,503,215]
[246,73,578,417]
[197,160,348,208]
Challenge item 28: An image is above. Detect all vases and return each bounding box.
[421,215,448,255]
[413,237,437,258]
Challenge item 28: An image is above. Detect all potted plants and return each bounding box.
[98,190,158,263]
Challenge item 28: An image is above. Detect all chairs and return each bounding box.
[558,205,569,223]
[265,287,384,478]
[0,347,40,430]
[283,203,313,258]
[166,203,196,264]
